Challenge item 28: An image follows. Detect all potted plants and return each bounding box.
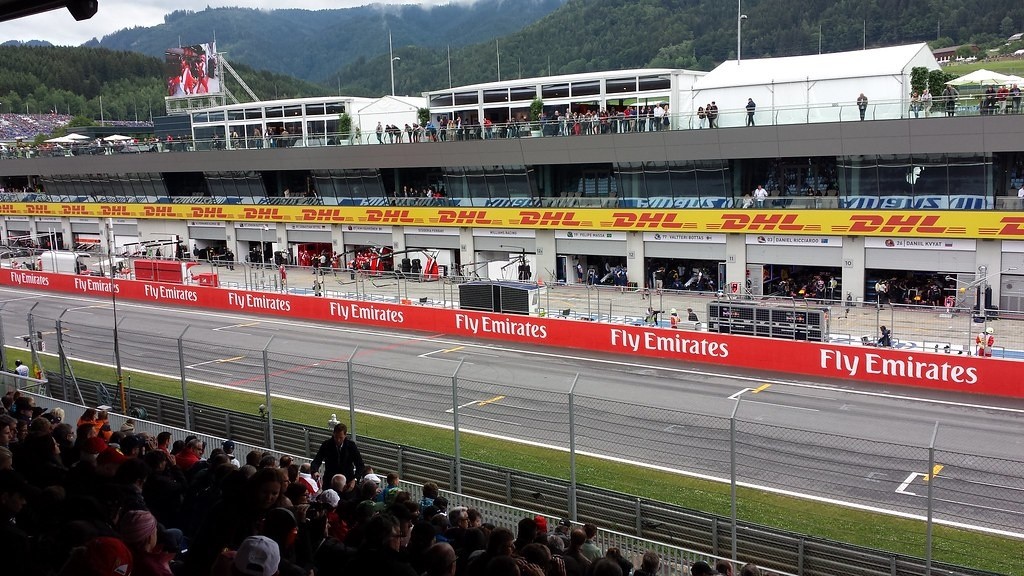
[338,113,351,145]
[530,96,544,137]
[908,67,961,118]
[418,107,430,142]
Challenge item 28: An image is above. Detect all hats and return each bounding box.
[323,489,340,507]
[97,447,129,464]
[535,516,547,532]
[123,509,158,542]
[365,474,382,484]
[33,407,48,416]
[85,537,132,576]
[559,518,573,527]
[234,535,280,575]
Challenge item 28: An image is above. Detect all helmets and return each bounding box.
[986,327,994,334]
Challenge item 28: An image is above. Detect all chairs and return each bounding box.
[559,309,570,319]
[862,337,874,347]
[420,297,427,305]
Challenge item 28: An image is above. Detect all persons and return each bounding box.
[247,245,383,296]
[1017,184,1024,208]
[0,184,45,194]
[875,326,891,348]
[976,327,994,357]
[976,84,1021,114]
[857,93,868,122]
[0,114,191,156]
[745,98,756,127]
[697,100,719,129]
[0,388,761,576]
[376,102,672,144]
[911,85,960,119]
[10,237,56,272]
[210,127,291,152]
[284,186,445,206]
[763,272,941,309]
[577,261,718,292]
[355,126,362,145]
[645,307,700,330]
[169,54,210,96]
[741,161,839,211]
[14,360,29,391]
[139,242,235,270]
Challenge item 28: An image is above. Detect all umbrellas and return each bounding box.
[103,135,132,144]
[1004,74,1024,104]
[64,132,90,141]
[945,69,1007,113]
[43,137,79,149]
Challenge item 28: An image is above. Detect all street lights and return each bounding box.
[738,0,748,66]
[728,386,756,576]
[389,27,400,96]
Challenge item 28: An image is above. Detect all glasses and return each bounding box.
[393,531,406,538]
[68,432,74,435]
